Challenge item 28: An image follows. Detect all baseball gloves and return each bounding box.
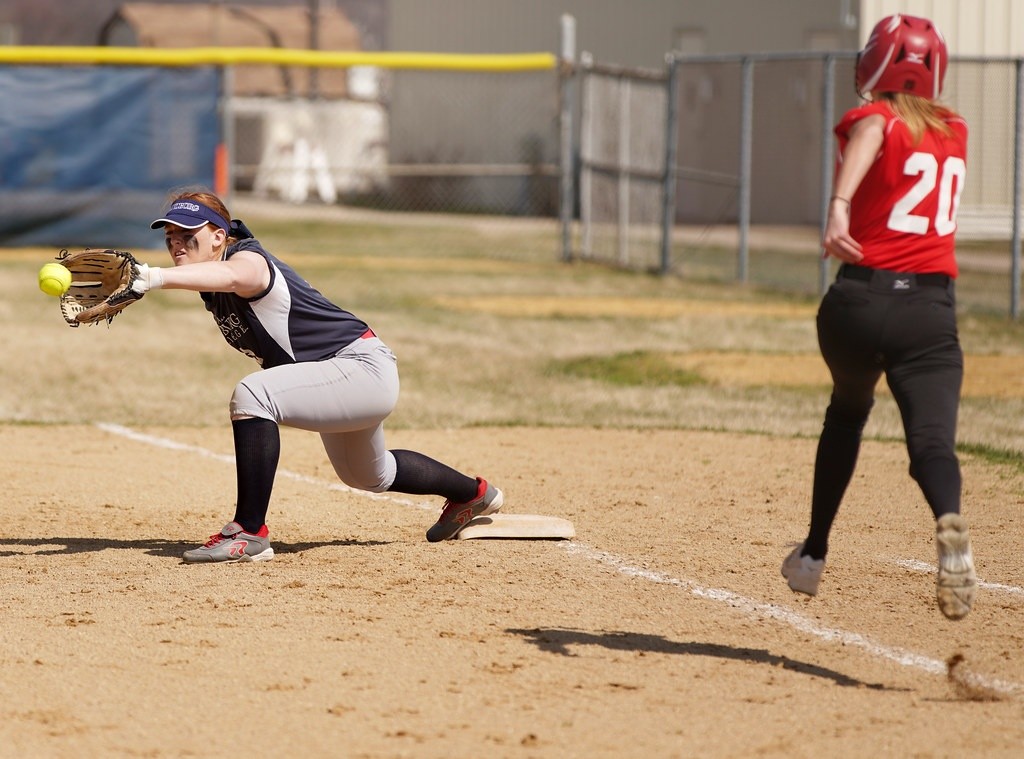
[55,248,145,329]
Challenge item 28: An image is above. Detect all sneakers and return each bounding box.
[782,541,823,596]
[935,512,975,620]
[183,522,275,562]
[426,477,503,540]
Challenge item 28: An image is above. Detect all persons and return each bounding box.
[780,9,976,624]
[127,185,508,564]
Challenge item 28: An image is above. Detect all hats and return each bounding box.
[150,199,231,236]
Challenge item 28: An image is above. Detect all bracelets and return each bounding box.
[828,195,854,207]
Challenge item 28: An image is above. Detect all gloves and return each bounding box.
[131,264,163,292]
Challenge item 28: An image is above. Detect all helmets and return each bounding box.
[858,15,948,99]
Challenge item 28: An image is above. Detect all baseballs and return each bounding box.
[38,262,72,296]
